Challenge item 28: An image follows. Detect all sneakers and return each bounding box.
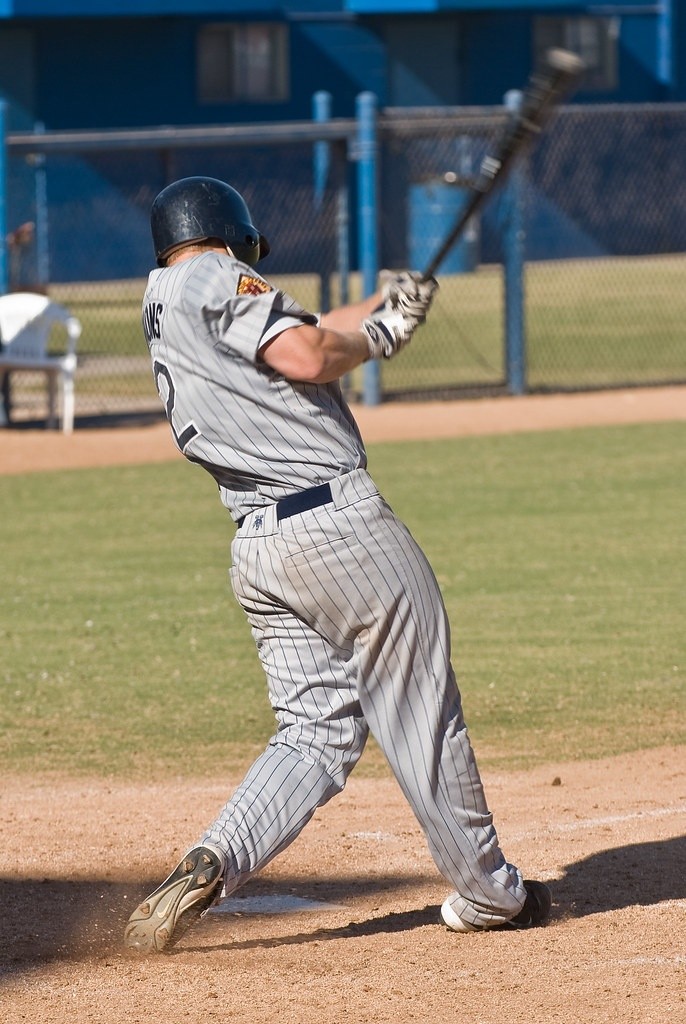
[438,880,552,933]
[124,845,226,957]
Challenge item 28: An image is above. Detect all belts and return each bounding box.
[237,482,333,529]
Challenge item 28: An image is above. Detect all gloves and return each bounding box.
[360,270,440,359]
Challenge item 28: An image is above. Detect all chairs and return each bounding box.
[0,292,82,434]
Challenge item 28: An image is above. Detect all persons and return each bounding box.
[123,176,550,954]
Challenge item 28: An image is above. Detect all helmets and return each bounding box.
[151,176,270,267]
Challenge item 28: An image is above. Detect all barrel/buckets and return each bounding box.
[408,173,480,276]
